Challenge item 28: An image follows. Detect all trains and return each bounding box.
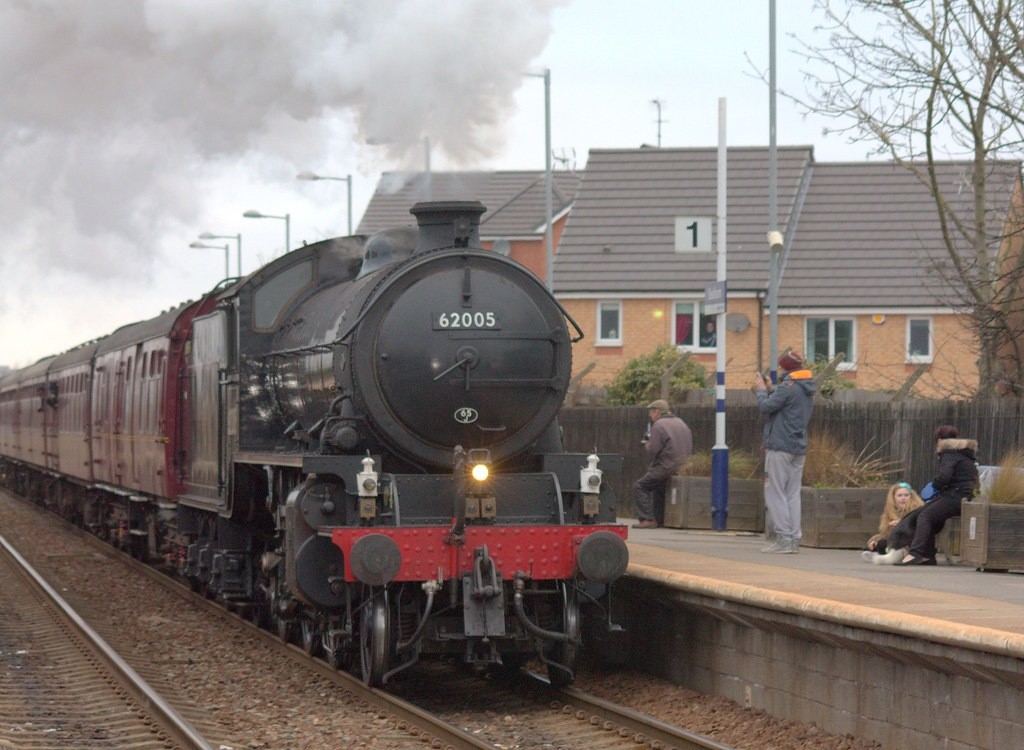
[0,199,630,693]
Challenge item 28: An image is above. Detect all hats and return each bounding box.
[936,426,958,438]
[778,351,802,370]
[647,400,669,410]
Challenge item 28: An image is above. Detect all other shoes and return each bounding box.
[894,552,937,566]
[862,549,902,564]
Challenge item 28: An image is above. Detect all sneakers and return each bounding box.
[761,541,800,554]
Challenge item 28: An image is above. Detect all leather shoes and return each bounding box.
[632,520,663,528]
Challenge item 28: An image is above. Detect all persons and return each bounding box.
[752,349,815,554]
[629,399,693,530]
[700,321,717,348]
[893,426,979,567]
[860,481,925,565]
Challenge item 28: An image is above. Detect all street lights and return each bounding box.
[297,171,351,236]
[243,210,290,254]
[365,133,433,201]
[199,232,241,278]
[189,242,229,284]
